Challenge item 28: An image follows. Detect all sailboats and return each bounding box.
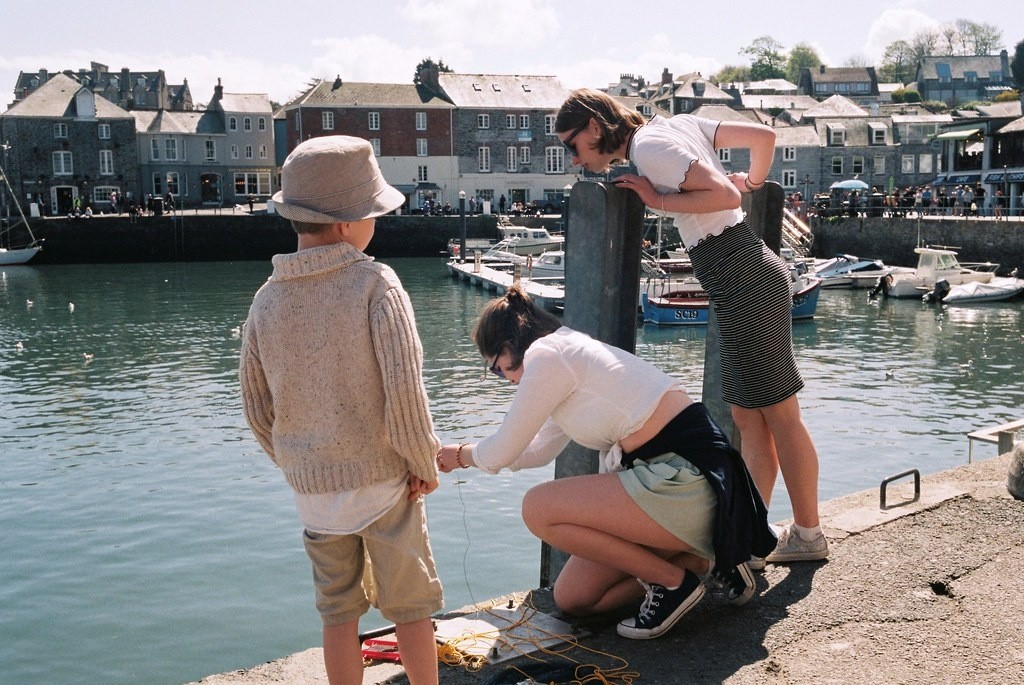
[0,161,45,265]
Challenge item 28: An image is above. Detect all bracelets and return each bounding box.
[745,178,760,190]
[456,443,472,468]
[662,193,666,218]
[748,175,767,185]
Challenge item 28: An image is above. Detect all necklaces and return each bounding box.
[624,124,640,160]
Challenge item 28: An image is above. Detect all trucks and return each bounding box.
[532,196,563,213]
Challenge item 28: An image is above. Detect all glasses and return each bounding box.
[561,119,592,157]
[489,338,515,378]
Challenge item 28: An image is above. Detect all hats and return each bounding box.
[271,135,405,223]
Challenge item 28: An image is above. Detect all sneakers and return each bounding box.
[707,556,756,604]
[765,523,829,561]
[616,567,706,639]
[747,554,766,569]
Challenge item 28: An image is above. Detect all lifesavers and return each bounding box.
[526,254,532,269]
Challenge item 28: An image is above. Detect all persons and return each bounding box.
[237,135,444,685]
[552,87,829,570]
[784,185,1005,222]
[399,193,540,218]
[33,190,255,218]
[436,284,779,641]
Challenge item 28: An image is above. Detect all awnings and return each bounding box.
[926,129,981,141]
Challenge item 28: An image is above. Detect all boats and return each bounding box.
[441,214,565,285]
[811,252,915,287]
[877,217,1000,298]
[914,269,1024,302]
[639,194,823,327]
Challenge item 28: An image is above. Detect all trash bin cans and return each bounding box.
[483,201,491,215]
[818,196,831,218]
[872,192,884,218]
[154,196,165,216]
[267,200,275,213]
[29,202,40,218]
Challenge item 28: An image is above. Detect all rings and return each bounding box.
[438,455,442,460]
